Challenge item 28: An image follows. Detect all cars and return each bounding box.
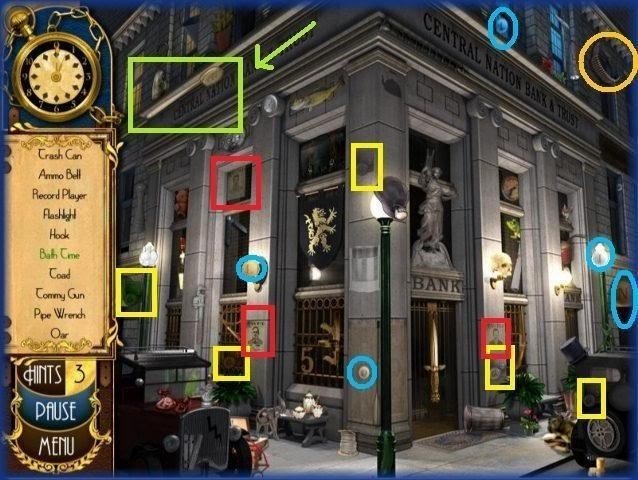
[115,346,252,479]
[570,347,638,468]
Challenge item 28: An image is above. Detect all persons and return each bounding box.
[248,327,263,346]
[417,166,460,254]
[489,328,502,345]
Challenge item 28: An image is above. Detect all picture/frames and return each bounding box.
[0,127,118,362]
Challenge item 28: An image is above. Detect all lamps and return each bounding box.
[554,267,573,296]
[490,252,512,289]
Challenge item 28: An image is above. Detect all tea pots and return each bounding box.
[292,391,328,421]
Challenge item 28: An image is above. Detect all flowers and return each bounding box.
[521,409,540,429]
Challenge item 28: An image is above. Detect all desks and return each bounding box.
[273,410,330,445]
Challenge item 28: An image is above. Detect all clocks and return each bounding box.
[10,33,101,122]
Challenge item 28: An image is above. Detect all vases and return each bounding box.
[524,429,534,436]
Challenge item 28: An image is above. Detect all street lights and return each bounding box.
[138,241,157,345]
[369,193,397,478]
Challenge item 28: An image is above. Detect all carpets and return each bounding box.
[414,430,508,453]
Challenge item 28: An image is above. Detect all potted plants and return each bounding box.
[500,374,543,420]
[210,381,256,416]
